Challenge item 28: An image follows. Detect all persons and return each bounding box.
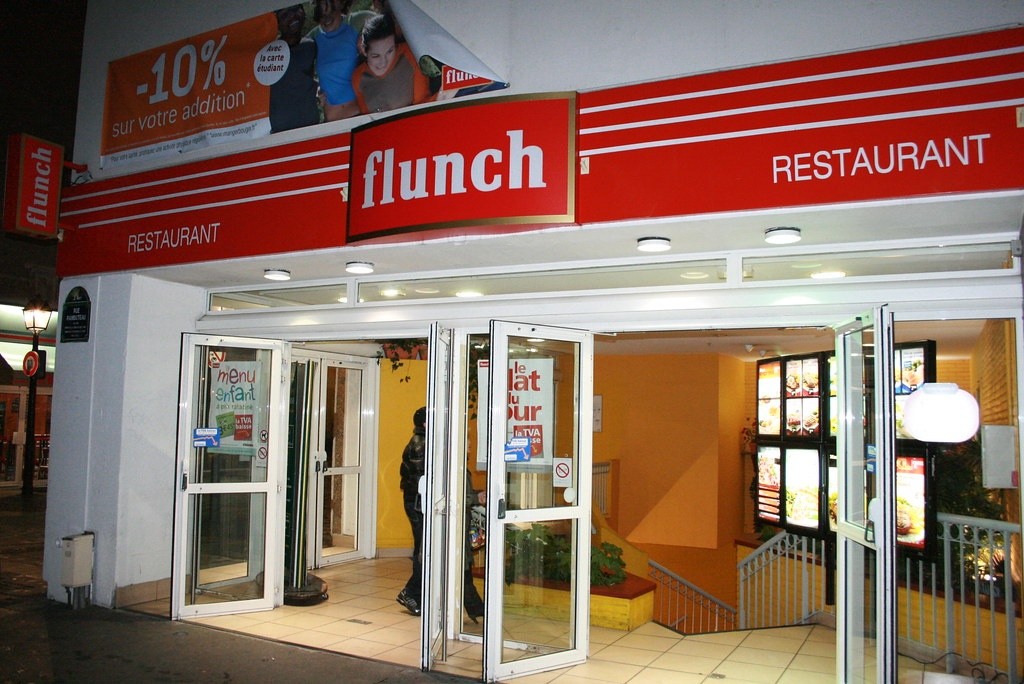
[269,3,320,136]
[396,407,434,616]
[420,427,486,624]
[352,14,458,117]
[305,0,366,123]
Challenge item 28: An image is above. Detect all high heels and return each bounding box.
[469,607,484,624]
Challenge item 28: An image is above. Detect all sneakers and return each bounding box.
[397,588,421,616]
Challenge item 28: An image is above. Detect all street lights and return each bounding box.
[21,298,51,510]
